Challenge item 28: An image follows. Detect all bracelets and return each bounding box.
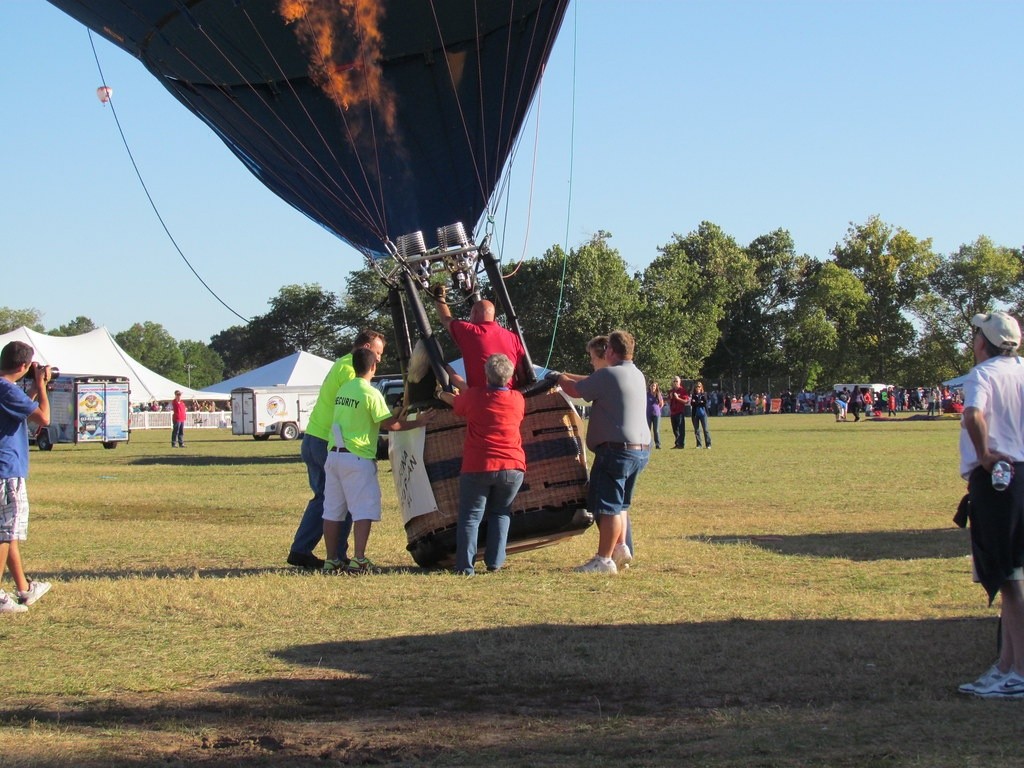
[438,391,445,400]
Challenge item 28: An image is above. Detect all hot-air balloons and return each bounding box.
[47,0,596,569]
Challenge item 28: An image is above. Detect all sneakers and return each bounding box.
[324,558,351,574]
[347,556,384,574]
[573,553,617,574]
[611,543,633,569]
[0,589,28,612]
[17,581,52,606]
[958,665,1024,697]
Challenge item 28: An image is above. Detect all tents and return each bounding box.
[0,324,336,403]
[942,375,964,387]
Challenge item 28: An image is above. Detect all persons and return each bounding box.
[429,282,525,393]
[172,390,187,448]
[832,384,964,423]
[287,330,386,569]
[690,382,712,449]
[0,341,52,614]
[647,381,663,449]
[957,312,1024,698]
[436,353,526,576]
[129,399,232,413]
[706,385,838,416]
[322,347,438,574]
[667,376,690,449]
[544,330,652,574]
[403,347,446,413]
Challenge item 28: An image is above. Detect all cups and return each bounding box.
[991,461,1011,491]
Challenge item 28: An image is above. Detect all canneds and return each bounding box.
[992,461,1010,490]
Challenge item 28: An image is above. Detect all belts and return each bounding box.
[600,442,650,452]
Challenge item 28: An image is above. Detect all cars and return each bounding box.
[374,379,404,411]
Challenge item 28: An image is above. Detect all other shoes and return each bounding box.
[172,445,178,448]
[707,446,711,449]
[287,551,325,568]
[656,445,661,449]
[696,446,702,449]
[180,444,186,447]
[670,446,684,450]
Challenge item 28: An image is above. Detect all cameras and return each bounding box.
[24,362,59,380]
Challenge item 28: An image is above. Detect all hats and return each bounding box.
[972,311,1023,363]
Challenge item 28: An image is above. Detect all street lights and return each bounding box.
[184,363,197,388]
